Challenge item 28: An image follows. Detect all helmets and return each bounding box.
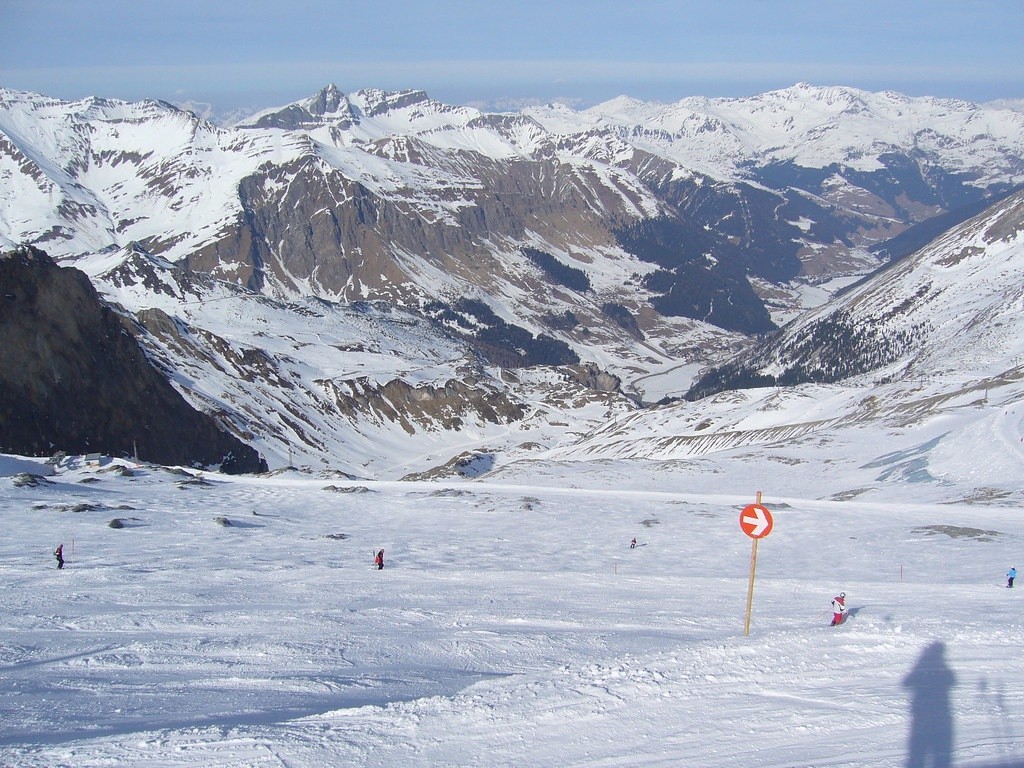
[840,592,845,597]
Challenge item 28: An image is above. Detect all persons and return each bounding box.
[56,544,65,570]
[630,538,636,548]
[1006,567,1016,588]
[830,592,848,626]
[377,549,384,569]
